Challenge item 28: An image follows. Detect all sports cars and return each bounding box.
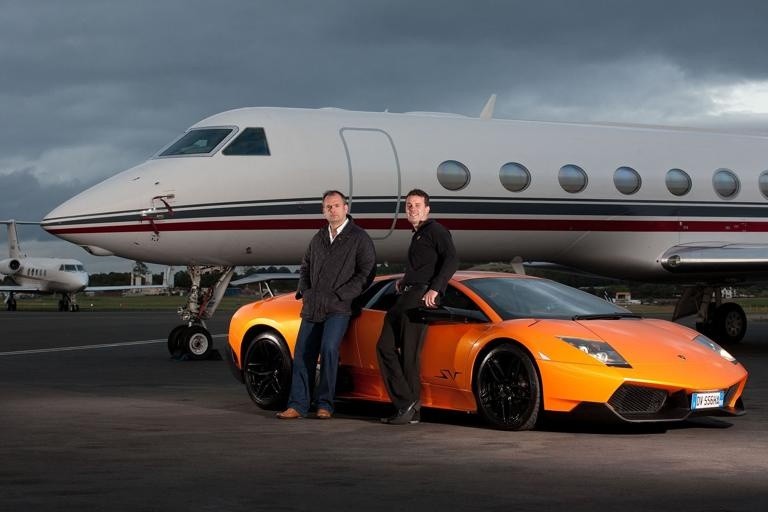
[225,265,752,439]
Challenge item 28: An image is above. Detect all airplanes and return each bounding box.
[0,218,172,314]
[38,90,768,363]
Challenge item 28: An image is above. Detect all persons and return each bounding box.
[376,189,457,424]
[276,190,376,418]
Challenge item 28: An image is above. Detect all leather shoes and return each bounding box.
[275,408,307,418]
[380,399,423,424]
[316,408,333,419]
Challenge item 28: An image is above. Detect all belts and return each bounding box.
[397,284,429,292]
